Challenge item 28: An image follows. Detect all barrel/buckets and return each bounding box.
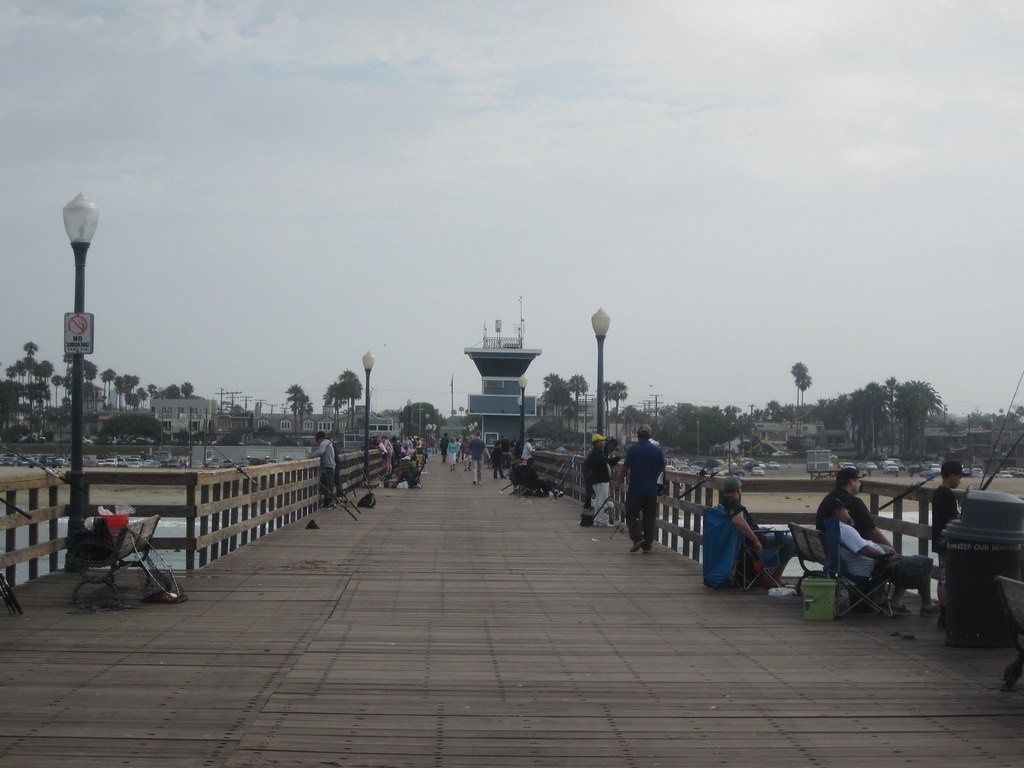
[99,512,129,544]
[801,578,838,621]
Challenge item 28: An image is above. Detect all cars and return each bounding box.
[83,434,245,447]
[0,453,296,469]
[246,439,271,446]
[665,457,780,476]
[771,451,794,457]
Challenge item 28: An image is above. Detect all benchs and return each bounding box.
[994,576,1024,692]
[65,514,162,603]
[788,522,830,596]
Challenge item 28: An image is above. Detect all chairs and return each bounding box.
[511,463,539,497]
[702,506,792,593]
[823,518,895,618]
[393,459,427,489]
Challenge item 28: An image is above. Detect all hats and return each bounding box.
[638,423,652,435]
[724,476,742,489]
[402,456,411,460]
[592,433,606,442]
[836,467,863,480]
[941,460,970,476]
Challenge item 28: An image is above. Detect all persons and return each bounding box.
[817,460,978,616]
[581,423,667,555]
[307,430,568,509]
[720,475,798,586]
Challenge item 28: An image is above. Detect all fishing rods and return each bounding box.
[301,446,362,515]
[675,405,820,501]
[977,371,1024,491]
[617,401,639,440]
[278,441,359,522]
[340,442,386,498]
[0,443,91,495]
[0,497,34,521]
[610,517,625,540]
[500,451,578,502]
[161,405,259,487]
[879,431,1012,512]
[593,490,615,519]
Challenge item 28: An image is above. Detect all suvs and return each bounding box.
[830,454,1024,479]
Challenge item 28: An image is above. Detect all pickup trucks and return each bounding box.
[19,434,46,442]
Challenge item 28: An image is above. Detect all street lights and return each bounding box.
[697,422,700,459]
[62,188,100,578]
[516,373,528,448]
[425,424,436,438]
[592,304,613,436]
[362,350,375,484]
[411,407,424,436]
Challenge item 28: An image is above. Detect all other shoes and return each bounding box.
[882,599,913,615]
[594,521,614,527]
[630,536,652,554]
[919,601,941,616]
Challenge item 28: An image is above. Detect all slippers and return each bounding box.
[768,582,796,590]
